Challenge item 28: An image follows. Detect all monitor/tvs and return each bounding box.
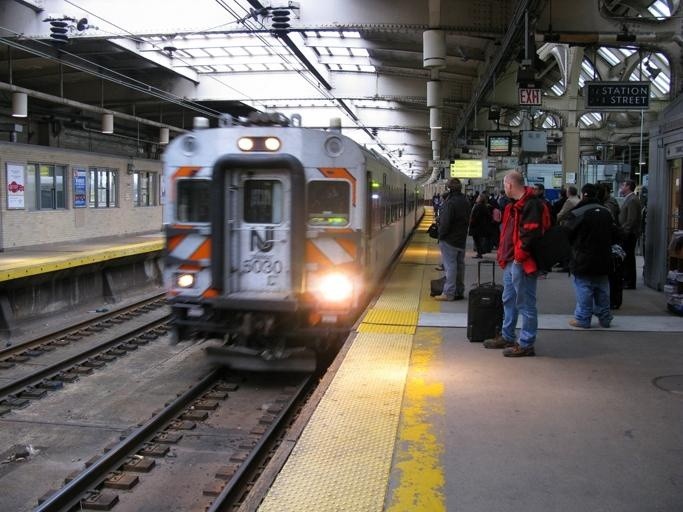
[487,136,512,156]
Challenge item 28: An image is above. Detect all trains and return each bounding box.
[162,125,424,379]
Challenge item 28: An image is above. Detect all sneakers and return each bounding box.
[483,336,515,349]
[568,319,590,328]
[434,264,444,270]
[502,345,535,357]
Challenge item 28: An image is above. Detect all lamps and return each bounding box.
[422,27,447,189]
[99,113,113,135]
[9,93,27,118]
[159,128,169,146]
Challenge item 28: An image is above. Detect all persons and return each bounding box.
[422,172,646,310]
[483,171,551,357]
[559,183,632,328]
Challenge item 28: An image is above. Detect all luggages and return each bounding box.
[466,260,504,342]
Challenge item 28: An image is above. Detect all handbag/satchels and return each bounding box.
[610,237,631,272]
[528,224,562,275]
[426,221,440,239]
[430,276,466,300]
